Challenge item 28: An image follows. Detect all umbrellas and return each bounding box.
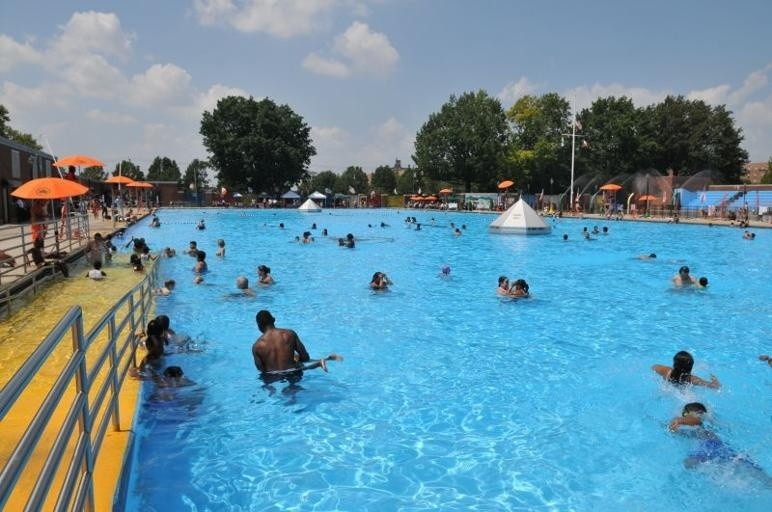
[53,153,103,176]
[637,195,657,201]
[105,175,154,202]
[599,183,620,204]
[9,176,90,232]
[439,188,452,200]
[496,180,515,188]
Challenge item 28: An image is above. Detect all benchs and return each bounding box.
[686,190,772,208]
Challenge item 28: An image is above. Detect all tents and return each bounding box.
[281,190,327,210]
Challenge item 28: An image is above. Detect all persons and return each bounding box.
[669,401,772,491]
[28,198,48,250]
[640,253,709,289]
[653,351,720,388]
[317,198,450,211]
[757,354,771,367]
[280,214,465,291]
[536,191,756,242]
[497,276,529,292]
[238,265,310,373]
[1,248,18,285]
[210,196,278,209]
[57,191,225,388]
[25,237,69,278]
